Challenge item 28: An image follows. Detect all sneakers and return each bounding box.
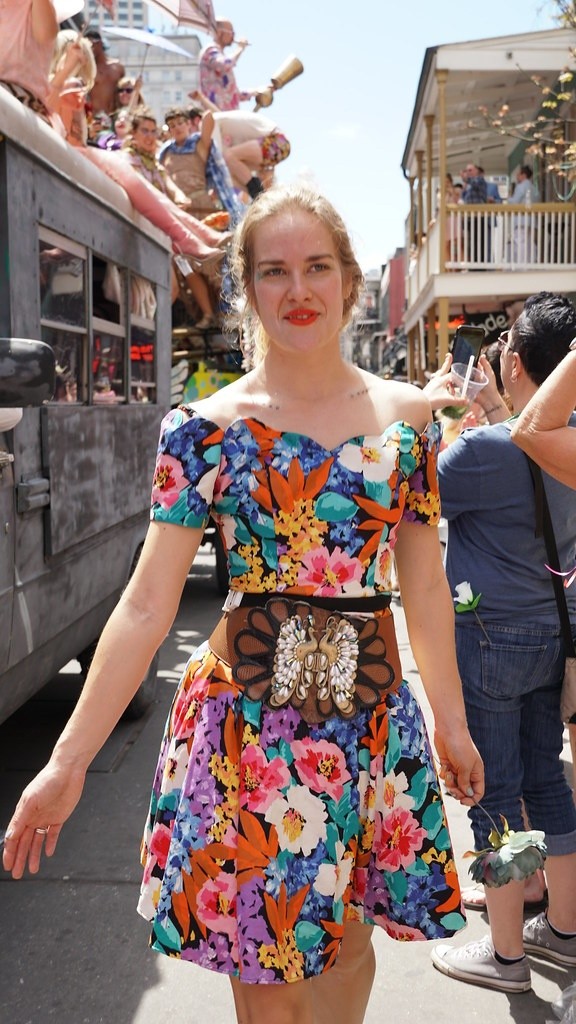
[522,908,576,967]
[430,934,531,993]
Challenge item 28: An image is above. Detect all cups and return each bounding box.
[440,362,490,420]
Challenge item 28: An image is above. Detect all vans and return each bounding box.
[0,80,174,721]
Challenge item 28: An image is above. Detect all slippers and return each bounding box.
[463,882,548,911]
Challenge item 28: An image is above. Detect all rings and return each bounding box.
[36,825,50,834]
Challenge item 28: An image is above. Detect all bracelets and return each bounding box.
[569,338,576,350]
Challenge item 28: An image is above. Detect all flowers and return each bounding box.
[434,756,548,888]
[453,582,493,645]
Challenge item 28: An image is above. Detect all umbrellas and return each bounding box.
[98,25,193,76]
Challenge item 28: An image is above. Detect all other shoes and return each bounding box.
[196,314,219,329]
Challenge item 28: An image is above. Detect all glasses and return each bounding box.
[496,330,518,357]
[118,88,134,94]
[221,28,234,35]
[90,38,101,44]
[138,127,159,137]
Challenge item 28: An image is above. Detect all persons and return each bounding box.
[1,186,486,1024]
[409,163,540,272]
[0,0,291,403]
[420,299,576,993]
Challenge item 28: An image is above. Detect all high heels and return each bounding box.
[211,231,234,246]
[172,241,227,280]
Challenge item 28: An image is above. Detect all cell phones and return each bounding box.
[448,325,485,380]
